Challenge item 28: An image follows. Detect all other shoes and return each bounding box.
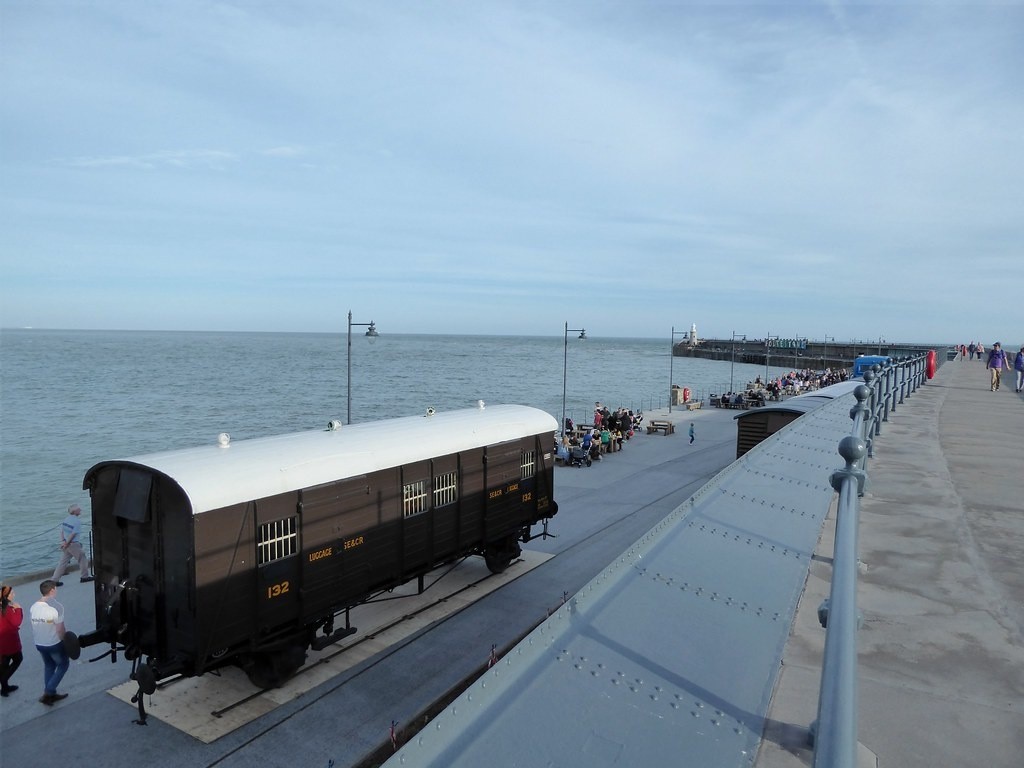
[80,577,95,583]
[56,582,63,587]
[51,693,68,702]
[0,685,19,697]
[39,693,54,706]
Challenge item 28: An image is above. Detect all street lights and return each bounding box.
[853,338,863,365]
[824,334,836,374]
[765,331,780,389]
[793,333,807,372]
[347,308,380,425]
[669,326,690,413]
[562,321,587,438]
[730,330,748,394]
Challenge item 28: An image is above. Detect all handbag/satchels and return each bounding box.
[629,428,634,436]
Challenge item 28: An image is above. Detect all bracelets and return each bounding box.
[62,540,66,542]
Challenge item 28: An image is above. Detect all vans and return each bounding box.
[852,356,891,379]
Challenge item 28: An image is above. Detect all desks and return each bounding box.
[576,438,601,454]
[567,430,587,442]
[556,445,579,453]
[608,437,618,453]
[649,420,672,434]
[745,400,758,408]
[576,424,595,437]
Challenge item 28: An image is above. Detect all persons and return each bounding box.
[29,580,68,706]
[721,367,854,410]
[954,339,1024,392]
[554,401,642,469]
[49,504,94,586]
[688,422,694,445]
[0,585,23,697]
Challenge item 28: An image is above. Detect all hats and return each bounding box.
[0,586,12,600]
[993,342,1001,346]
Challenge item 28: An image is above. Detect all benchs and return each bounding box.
[686,402,701,410]
[646,426,669,436]
[554,455,565,464]
[654,424,676,434]
[742,379,833,399]
[722,403,745,410]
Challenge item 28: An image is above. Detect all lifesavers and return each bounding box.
[962,346,967,356]
[926,349,936,379]
